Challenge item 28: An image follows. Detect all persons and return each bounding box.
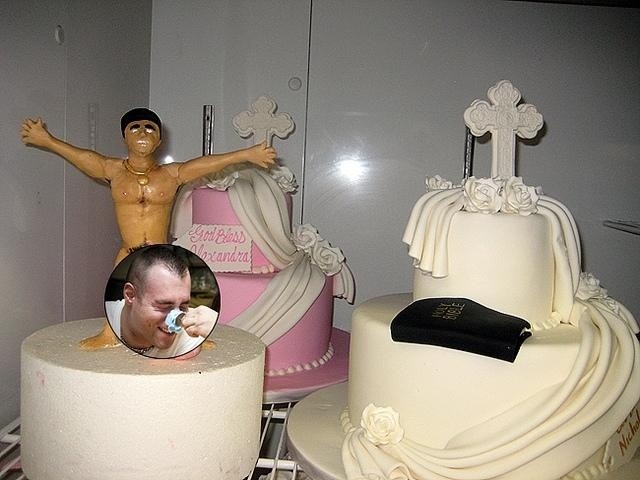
[106,249,218,358]
[19,107,274,352]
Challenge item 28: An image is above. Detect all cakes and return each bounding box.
[18,108,277,480]
[287,80,640,480]
[165,95,356,405]
